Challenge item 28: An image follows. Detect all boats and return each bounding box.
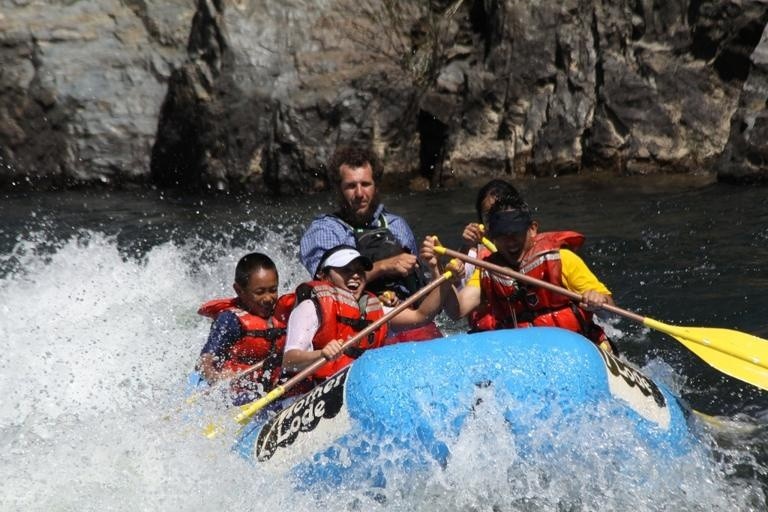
[178,323,708,497]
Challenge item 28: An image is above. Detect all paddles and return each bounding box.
[204,260,459,442]
[431,246,767,391]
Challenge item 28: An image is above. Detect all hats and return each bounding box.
[321,248,374,270]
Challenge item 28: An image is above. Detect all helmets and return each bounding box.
[488,196,531,234]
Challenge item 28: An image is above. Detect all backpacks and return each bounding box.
[341,214,427,308]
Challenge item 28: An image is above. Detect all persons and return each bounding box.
[299,154,443,346]
[420,178,616,354]
[200,246,466,403]
